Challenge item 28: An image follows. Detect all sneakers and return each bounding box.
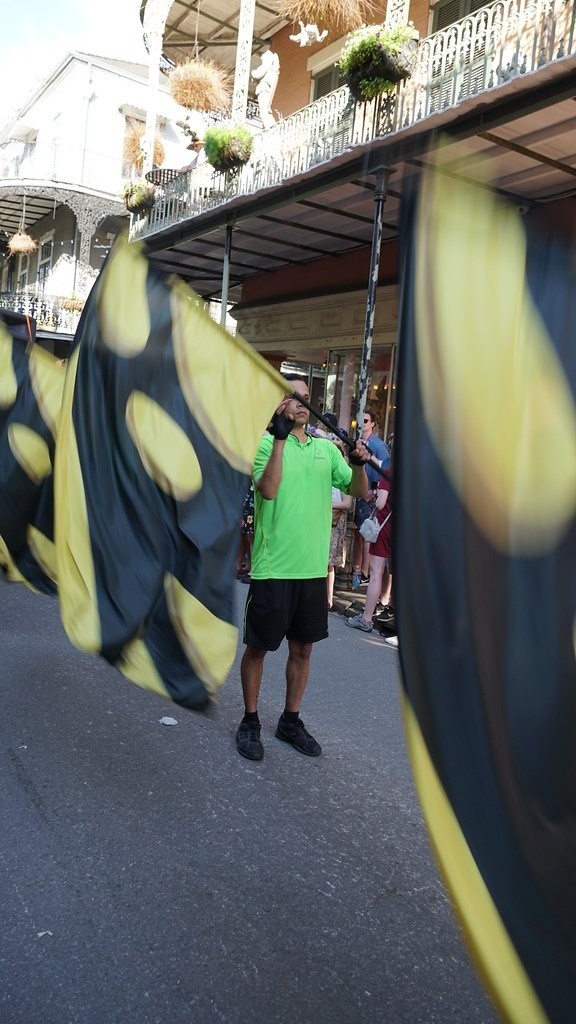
[352,578,369,586]
[377,606,396,621]
[345,616,373,632]
[236,721,264,761]
[372,603,388,617]
[275,718,322,756]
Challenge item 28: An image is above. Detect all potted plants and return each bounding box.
[201,118,253,173]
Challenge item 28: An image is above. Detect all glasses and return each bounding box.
[364,419,373,423]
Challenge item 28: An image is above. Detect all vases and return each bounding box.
[126,193,154,215]
[346,39,419,102]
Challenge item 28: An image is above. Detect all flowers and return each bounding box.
[117,177,152,207]
[334,21,419,98]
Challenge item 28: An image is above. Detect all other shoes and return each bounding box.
[384,636,398,645]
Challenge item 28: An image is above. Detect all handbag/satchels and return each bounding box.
[359,517,380,543]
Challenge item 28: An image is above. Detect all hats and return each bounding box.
[323,413,337,425]
[187,140,206,150]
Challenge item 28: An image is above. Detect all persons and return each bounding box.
[236,373,373,761]
[251,43,280,127]
[309,410,398,638]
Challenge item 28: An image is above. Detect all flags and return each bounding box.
[1,312,69,601]
[51,221,296,718]
[397,145,576,1024]
